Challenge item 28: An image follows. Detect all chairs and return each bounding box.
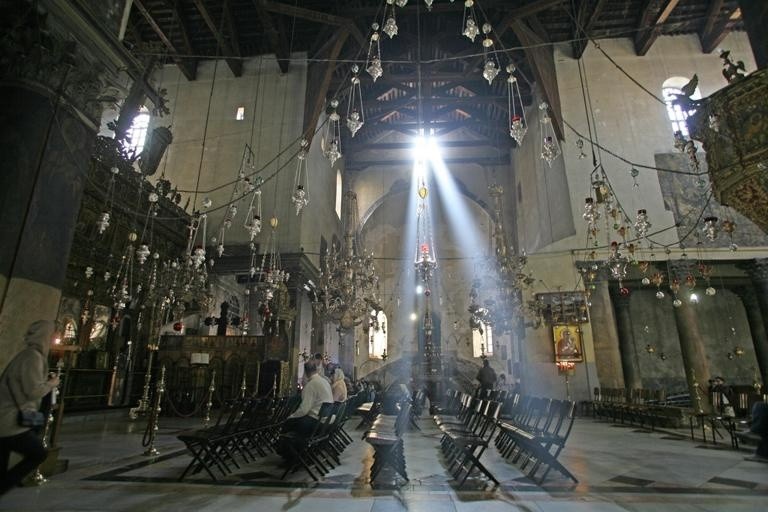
[578,387,673,431]
[690,390,764,452]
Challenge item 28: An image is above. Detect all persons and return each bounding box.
[268,350,508,471]
[713,376,736,417]
[711,379,721,415]
[707,378,715,405]
[555,327,580,360]
[1,318,62,498]
[738,399,768,462]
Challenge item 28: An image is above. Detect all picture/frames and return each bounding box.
[551,323,583,363]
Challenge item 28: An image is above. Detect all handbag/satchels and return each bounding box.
[16,407,46,428]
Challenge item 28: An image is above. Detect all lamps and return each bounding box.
[467,180,548,336]
[303,179,385,330]
